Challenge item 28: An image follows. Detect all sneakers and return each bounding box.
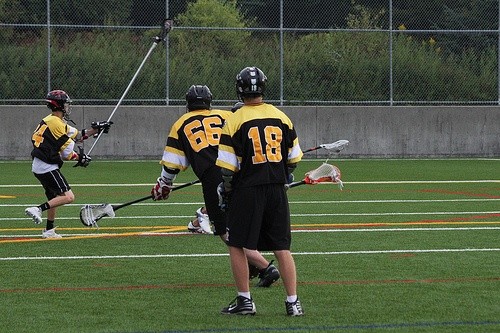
[285,295,305,317]
[25,207,43,225]
[221,296,256,315]
[42,226,62,239]
[195,206,213,236]
[188,220,204,233]
[260,260,279,288]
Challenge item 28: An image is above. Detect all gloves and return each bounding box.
[151,178,173,201]
[92,120,114,134]
[220,192,232,214]
[78,153,91,166]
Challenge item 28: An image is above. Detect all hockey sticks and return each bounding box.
[86,15,174,156]
[303,138,350,155]
[79,176,200,227]
[288,164,343,191]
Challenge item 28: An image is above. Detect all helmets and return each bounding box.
[230,101,244,113]
[185,84,213,110]
[46,90,72,120]
[235,66,267,101]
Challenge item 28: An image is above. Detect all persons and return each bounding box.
[150,85,280,288]
[25,90,113,239]
[215,67,304,316]
[188,102,244,234]
